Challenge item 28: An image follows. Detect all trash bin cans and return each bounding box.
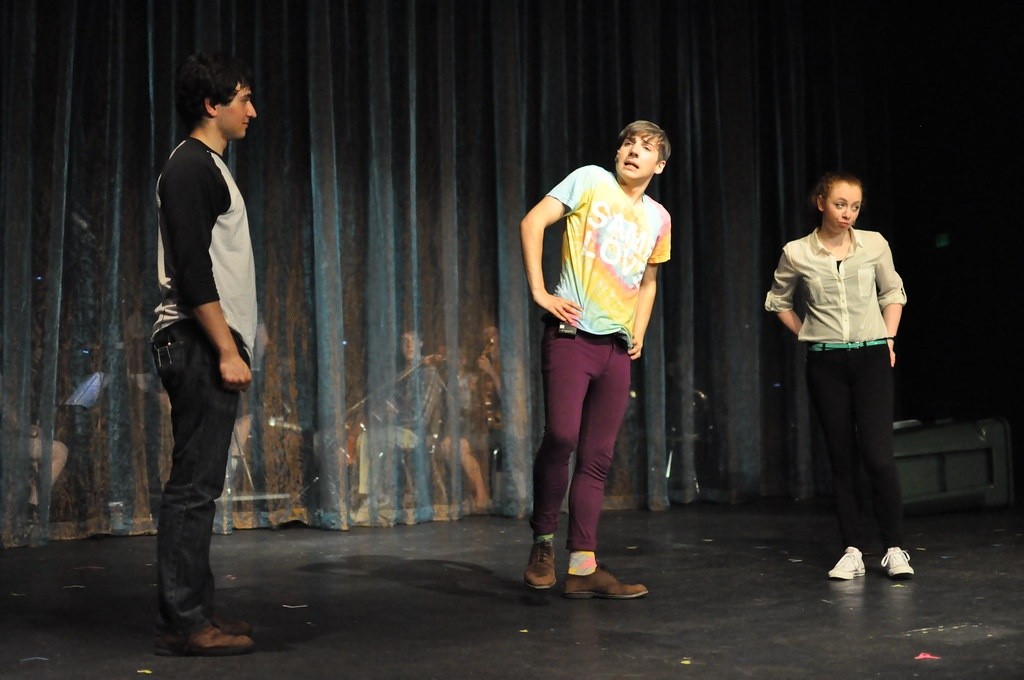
[892,415,1016,517]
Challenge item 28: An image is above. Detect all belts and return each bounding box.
[811,339,890,349]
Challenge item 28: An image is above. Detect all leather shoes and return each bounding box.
[525,537,557,605]
[566,565,648,599]
[152,616,254,656]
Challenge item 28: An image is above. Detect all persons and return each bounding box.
[520,119,671,600]
[1,297,174,532]
[212,311,500,526]
[148,52,258,657]
[764,172,915,583]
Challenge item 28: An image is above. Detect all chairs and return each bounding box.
[393,419,449,507]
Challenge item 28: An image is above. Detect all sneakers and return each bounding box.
[881,547,915,577]
[827,545,865,578]
